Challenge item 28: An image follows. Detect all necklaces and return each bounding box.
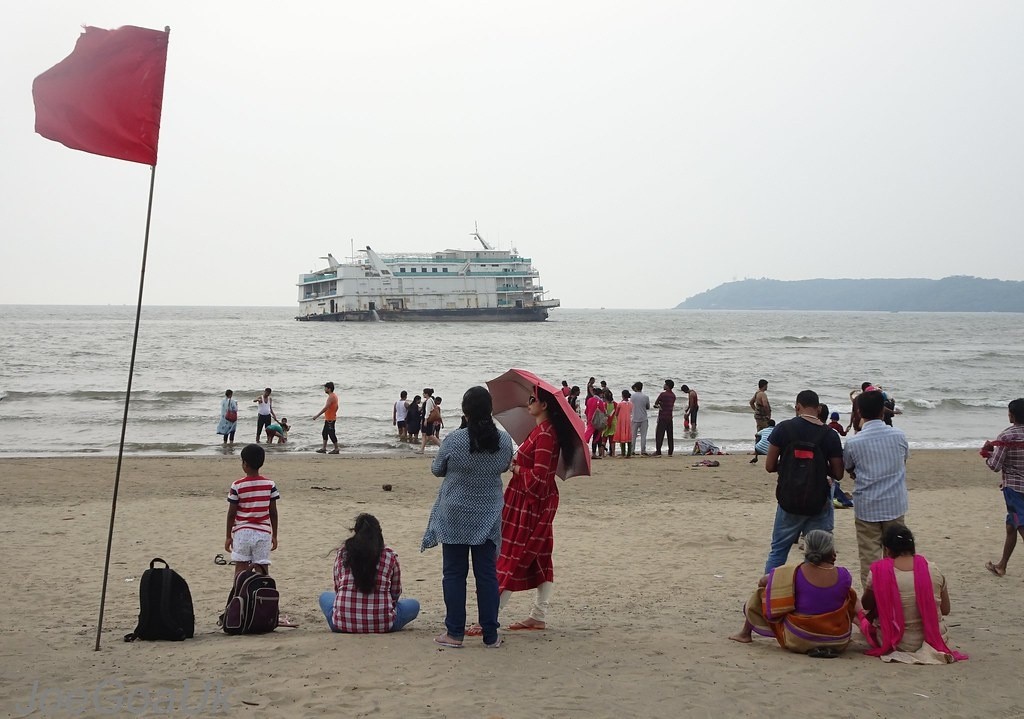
[798,415,819,420]
[810,562,836,570]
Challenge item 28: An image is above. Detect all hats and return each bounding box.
[829,413,840,420]
[864,382,884,393]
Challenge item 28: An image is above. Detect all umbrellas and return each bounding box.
[486,369,591,481]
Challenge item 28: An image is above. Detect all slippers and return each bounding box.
[985,561,1006,578]
[432,632,464,648]
[504,620,546,630]
[463,624,486,637]
[481,627,503,648]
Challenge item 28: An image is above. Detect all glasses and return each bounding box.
[527,395,538,405]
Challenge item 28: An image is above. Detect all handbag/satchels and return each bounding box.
[590,399,606,431]
[427,406,441,425]
[224,409,238,423]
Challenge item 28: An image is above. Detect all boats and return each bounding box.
[294,220,560,323]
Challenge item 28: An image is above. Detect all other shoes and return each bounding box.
[590,451,673,458]
[315,449,327,453]
[413,450,424,454]
[328,449,339,454]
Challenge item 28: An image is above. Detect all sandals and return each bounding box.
[213,553,227,566]
[278,612,300,627]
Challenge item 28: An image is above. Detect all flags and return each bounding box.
[33,26,169,165]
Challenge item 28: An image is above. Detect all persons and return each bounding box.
[748,380,902,466]
[985,398,1024,578]
[561,377,698,459]
[319,512,421,633]
[216,382,445,455]
[464,386,575,636]
[420,387,513,648]
[764,390,844,577]
[842,392,909,589]
[225,445,279,576]
[861,523,951,651]
[729,530,857,653]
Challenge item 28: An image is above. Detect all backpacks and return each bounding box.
[774,420,833,515]
[121,558,195,642]
[214,564,281,635]
